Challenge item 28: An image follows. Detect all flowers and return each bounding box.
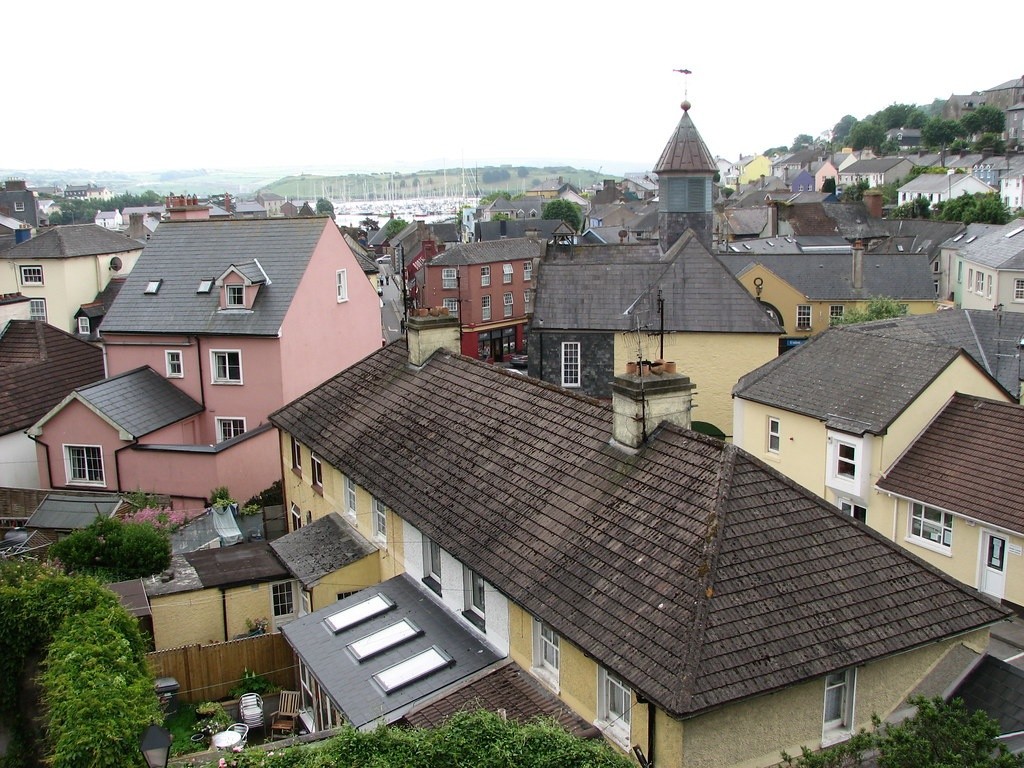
[191,718,225,735]
[227,667,278,699]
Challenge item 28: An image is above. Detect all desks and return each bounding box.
[212,731,242,752]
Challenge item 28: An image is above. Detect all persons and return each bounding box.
[400,318,405,335]
[379,273,390,285]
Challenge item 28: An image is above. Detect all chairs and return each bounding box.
[270,690,300,741]
[237,693,267,738]
[226,723,249,745]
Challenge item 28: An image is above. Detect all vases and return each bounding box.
[191,734,204,742]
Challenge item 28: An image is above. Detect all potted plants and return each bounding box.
[195,701,229,722]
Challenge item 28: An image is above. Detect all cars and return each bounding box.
[375,254,394,263]
[509,350,529,365]
